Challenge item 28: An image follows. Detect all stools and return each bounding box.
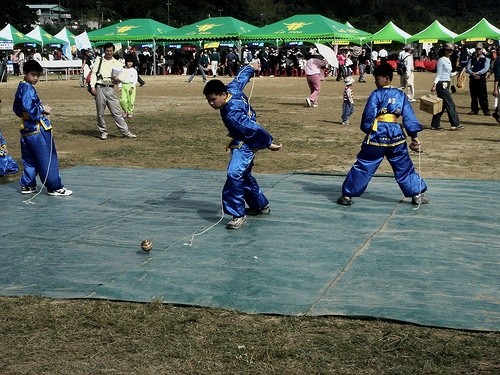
[261,67,305,76]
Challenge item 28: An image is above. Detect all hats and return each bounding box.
[310,48,320,55]
[344,77,355,85]
[445,43,454,51]
[405,45,414,50]
[476,43,483,48]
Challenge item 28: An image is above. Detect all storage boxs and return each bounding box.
[420,91,444,116]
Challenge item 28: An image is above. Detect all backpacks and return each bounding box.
[396,55,412,75]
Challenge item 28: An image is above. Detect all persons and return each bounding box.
[13,59,73,196]
[430,44,465,131]
[337,62,430,206]
[0,43,500,119]
[202,60,283,228]
[0,130,19,177]
[341,77,356,125]
[90,44,138,140]
[493,82,500,124]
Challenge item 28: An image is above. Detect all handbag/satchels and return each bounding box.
[451,73,456,93]
[88,81,96,96]
[365,61,370,74]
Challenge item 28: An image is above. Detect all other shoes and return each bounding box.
[358,80,366,83]
[139,83,145,87]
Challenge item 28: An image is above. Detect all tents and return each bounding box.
[87,19,177,75]
[369,21,413,49]
[407,19,459,44]
[0,19,93,55]
[240,14,372,77]
[156,16,261,68]
[454,17,500,43]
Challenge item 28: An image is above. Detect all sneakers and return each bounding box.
[124,133,137,138]
[306,98,318,107]
[468,110,500,125]
[336,196,352,204]
[226,204,270,229]
[259,75,274,78]
[123,112,132,118]
[342,121,350,125]
[187,80,208,83]
[21,186,73,196]
[413,193,430,204]
[408,99,416,101]
[430,124,464,130]
[101,132,108,139]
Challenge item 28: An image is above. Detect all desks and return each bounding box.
[37,60,83,81]
[377,59,437,71]
